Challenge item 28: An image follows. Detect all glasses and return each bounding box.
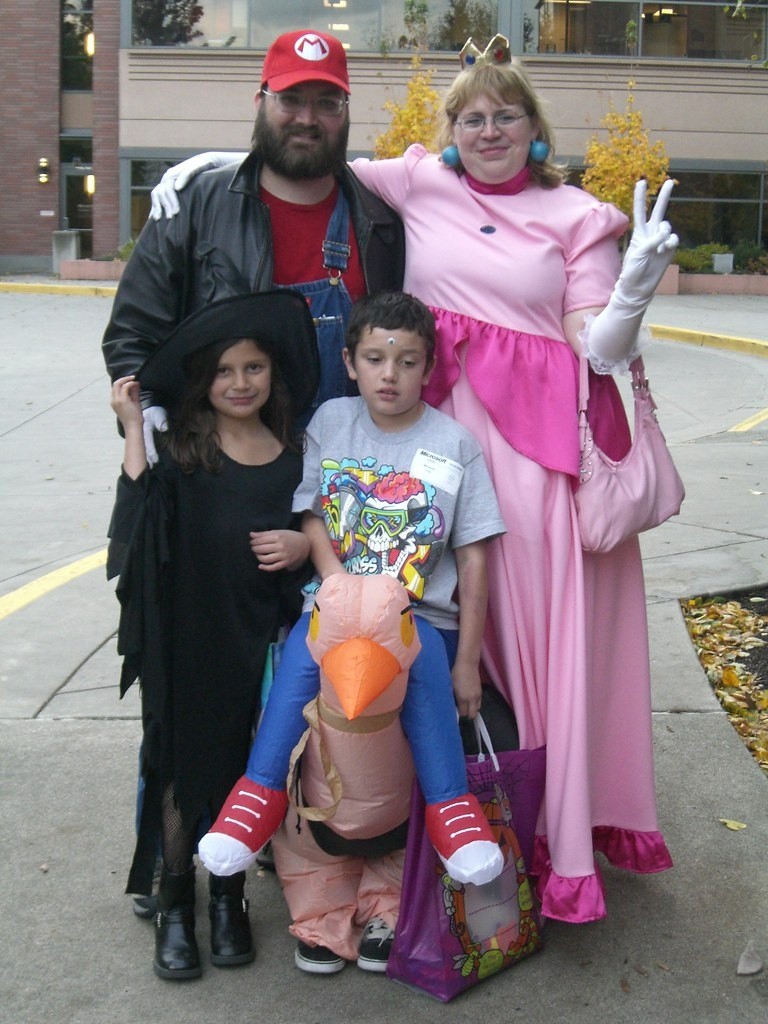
[455,113,528,133]
[261,89,349,116]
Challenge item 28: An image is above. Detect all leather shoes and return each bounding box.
[133,896,155,919]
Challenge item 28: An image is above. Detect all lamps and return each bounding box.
[39,157,52,183]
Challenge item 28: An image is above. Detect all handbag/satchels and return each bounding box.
[576,337,684,553]
[386,709,547,1004]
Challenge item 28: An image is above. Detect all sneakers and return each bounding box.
[294,939,346,973]
[356,916,397,971]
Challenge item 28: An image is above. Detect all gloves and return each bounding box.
[582,181,680,377]
[142,406,169,469]
[148,151,249,221]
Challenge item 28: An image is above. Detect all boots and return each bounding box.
[207,870,258,965]
[152,865,203,980]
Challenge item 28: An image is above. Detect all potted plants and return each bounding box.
[575,75,680,296]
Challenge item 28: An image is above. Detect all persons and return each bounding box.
[148,33,687,921]
[104,286,322,980]
[100,30,404,918]
[197,291,505,885]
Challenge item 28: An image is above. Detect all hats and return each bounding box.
[133,242,322,426]
[260,30,350,96]
